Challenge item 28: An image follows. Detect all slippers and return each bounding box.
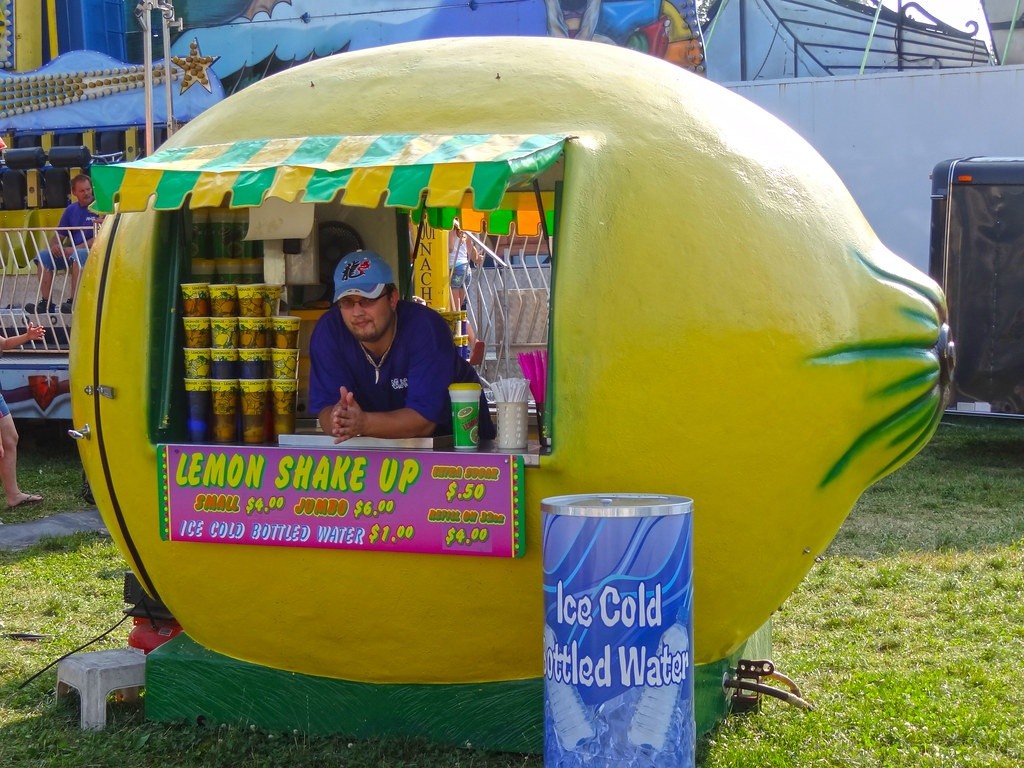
[9,493,44,509]
[469,341,485,365]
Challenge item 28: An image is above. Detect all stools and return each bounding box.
[56,648,146,733]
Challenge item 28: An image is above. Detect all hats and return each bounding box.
[333,249,394,304]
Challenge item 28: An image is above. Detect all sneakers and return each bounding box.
[24,297,56,314]
[60,299,73,313]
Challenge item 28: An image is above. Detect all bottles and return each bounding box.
[542,612,599,752]
[627,606,693,754]
[448,383,482,450]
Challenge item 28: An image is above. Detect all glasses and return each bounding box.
[337,291,389,309]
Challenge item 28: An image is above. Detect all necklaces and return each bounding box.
[358,329,395,385]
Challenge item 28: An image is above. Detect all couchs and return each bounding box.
[0,144,124,208]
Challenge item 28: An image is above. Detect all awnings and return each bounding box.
[395,192,556,238]
[88,136,565,217]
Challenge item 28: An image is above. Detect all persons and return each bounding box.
[308,249,496,445]
[0,322,45,509]
[449,220,483,353]
[24,174,99,313]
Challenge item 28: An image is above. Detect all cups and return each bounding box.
[179,280,301,417]
